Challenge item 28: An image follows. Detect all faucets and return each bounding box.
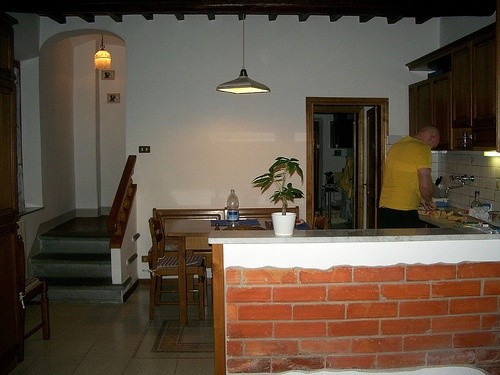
[445,174,474,194]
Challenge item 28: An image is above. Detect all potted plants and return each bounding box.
[253,155,305,235]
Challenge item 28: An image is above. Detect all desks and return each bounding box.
[147,241,211,267]
[321,188,340,228]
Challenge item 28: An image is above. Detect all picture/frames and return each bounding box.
[101,69,115,80]
[107,93,121,104]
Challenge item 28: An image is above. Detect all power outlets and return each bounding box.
[139,146,150,154]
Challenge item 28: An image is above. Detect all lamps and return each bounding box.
[93,34,112,71]
[217,14,271,94]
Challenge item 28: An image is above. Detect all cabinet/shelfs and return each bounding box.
[0,12,24,375]
[403,20,500,152]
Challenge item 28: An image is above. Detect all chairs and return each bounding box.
[146,217,207,319]
[177,235,212,321]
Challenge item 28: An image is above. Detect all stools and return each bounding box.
[24,277,52,343]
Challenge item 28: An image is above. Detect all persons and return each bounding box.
[379,126,441,228]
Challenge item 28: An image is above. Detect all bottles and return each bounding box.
[226,189,239,229]
[463,132,469,147]
[471,191,481,209]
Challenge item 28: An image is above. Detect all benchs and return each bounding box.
[148,206,299,239]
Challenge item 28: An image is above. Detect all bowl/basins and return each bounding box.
[487,210,500,228]
